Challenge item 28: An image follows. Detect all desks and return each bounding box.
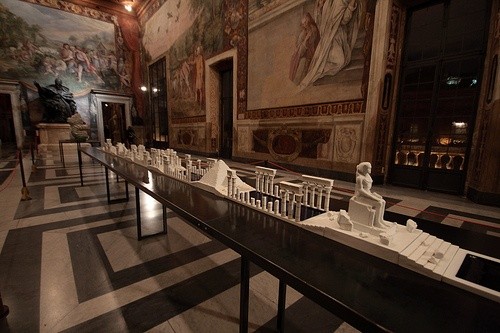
[76,146,499,333]
[58,140,103,165]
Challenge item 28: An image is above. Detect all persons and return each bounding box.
[354,162,392,229]
